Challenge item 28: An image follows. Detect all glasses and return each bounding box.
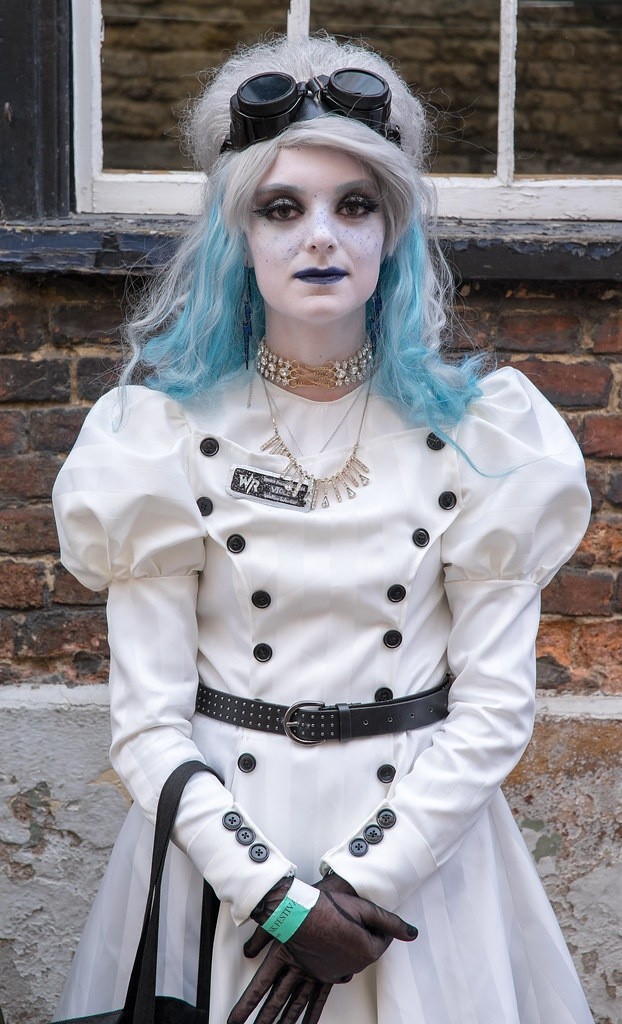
[216,69,399,151]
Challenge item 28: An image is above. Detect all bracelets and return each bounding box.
[262,876,320,944]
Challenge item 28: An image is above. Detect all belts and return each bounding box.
[191,687,456,745]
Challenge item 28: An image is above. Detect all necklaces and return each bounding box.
[253,333,376,510]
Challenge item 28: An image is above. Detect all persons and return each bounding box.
[52,26,596,1024]
